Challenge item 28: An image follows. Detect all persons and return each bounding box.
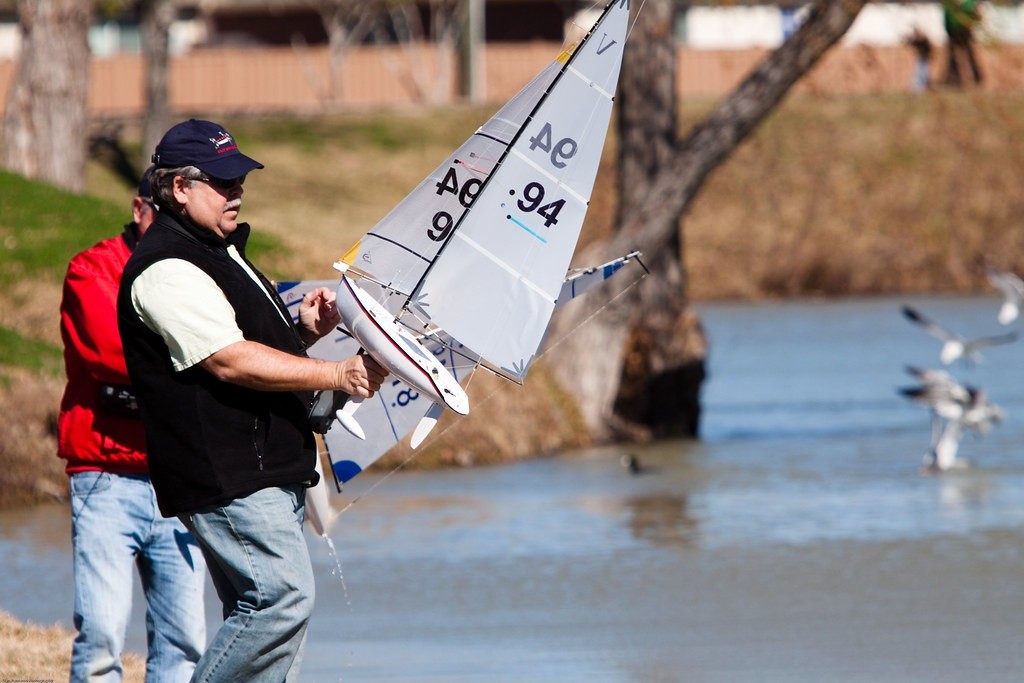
[55,166,211,683]
[117,117,390,683]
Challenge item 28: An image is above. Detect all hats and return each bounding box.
[139,166,161,210]
[151,119,265,179]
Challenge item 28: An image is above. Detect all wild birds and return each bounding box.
[882,252,1023,474]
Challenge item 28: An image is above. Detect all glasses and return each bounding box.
[182,176,244,189]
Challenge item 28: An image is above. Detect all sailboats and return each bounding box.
[273,247,652,543]
[330,1,633,419]
